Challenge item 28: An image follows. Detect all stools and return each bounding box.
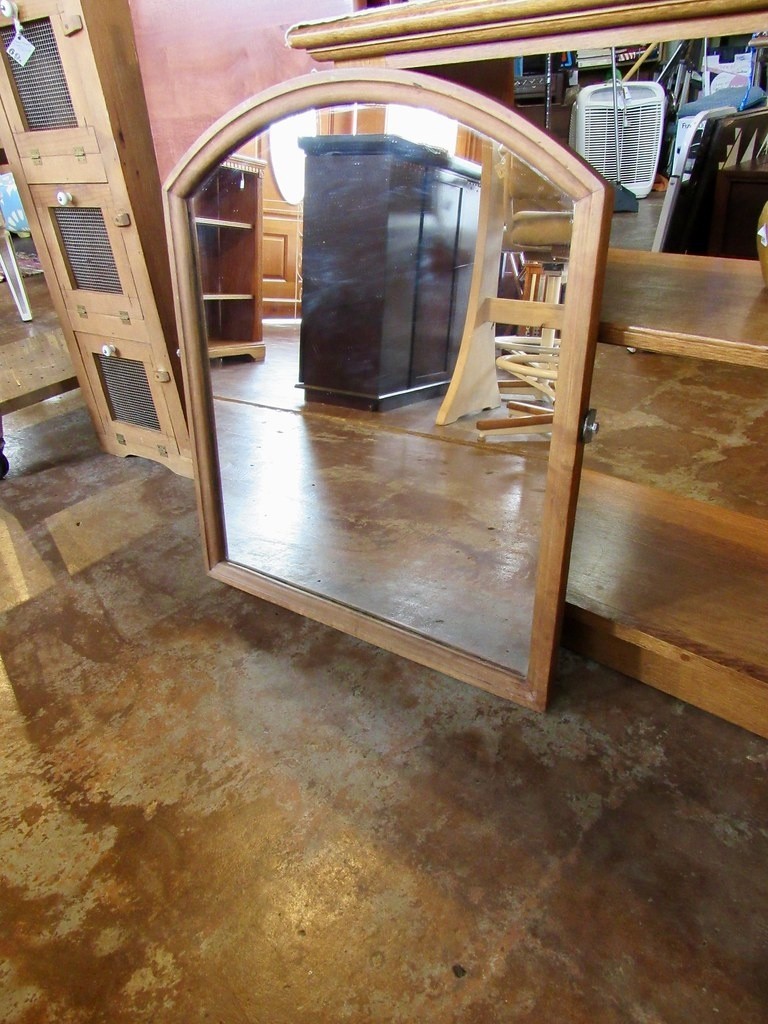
[0,173,39,321]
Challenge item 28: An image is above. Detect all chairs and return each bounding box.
[436,138,573,427]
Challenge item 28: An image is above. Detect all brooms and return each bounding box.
[608,47,640,214]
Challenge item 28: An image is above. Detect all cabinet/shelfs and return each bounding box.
[557,244,767,742]
[707,155,768,259]
[0,0,195,483]
[191,152,266,363]
[294,134,483,412]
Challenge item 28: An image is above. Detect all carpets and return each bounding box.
[0,248,44,284]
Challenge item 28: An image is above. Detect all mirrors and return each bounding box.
[162,68,614,714]
